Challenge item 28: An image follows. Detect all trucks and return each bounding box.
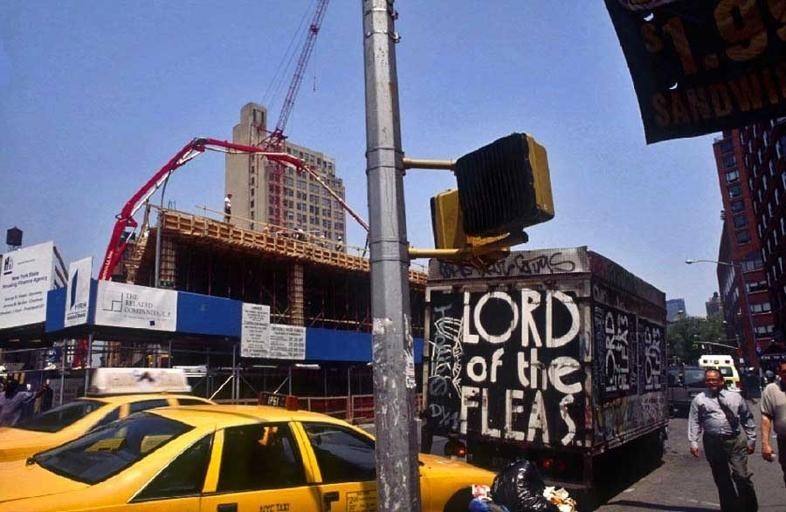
[668,350,744,420]
[419,247,676,508]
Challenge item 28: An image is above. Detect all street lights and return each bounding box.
[685,258,764,396]
[676,309,741,360]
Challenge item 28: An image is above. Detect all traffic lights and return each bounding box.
[446,130,549,246]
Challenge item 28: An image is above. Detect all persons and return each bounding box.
[0,379,50,426]
[688,369,759,511]
[760,359,786,487]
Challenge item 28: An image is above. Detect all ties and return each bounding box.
[713,393,739,432]
